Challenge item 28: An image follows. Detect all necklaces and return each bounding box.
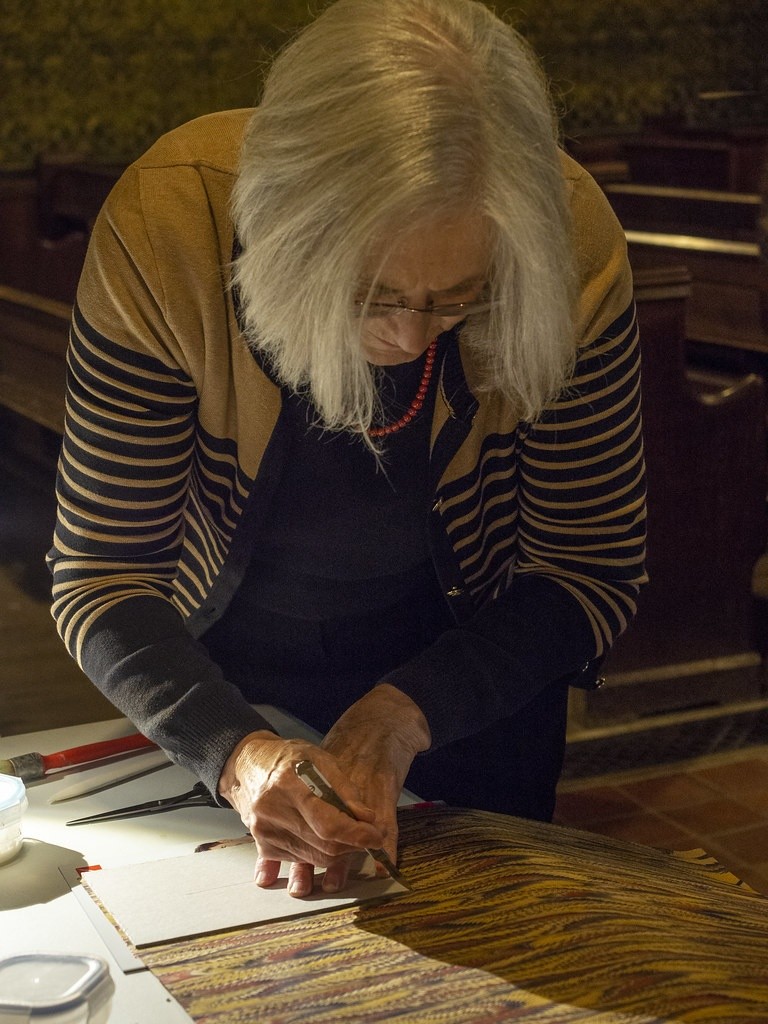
[368,337,436,437]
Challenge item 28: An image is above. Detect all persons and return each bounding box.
[47,0,647,895]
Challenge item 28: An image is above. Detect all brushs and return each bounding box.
[0,723,158,785]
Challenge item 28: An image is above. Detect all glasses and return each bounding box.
[352,295,491,317]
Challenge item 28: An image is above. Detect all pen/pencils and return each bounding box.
[284,751,415,906]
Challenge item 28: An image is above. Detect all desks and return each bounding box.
[0,703,424,1024]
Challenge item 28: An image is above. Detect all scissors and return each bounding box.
[49,775,228,835]
